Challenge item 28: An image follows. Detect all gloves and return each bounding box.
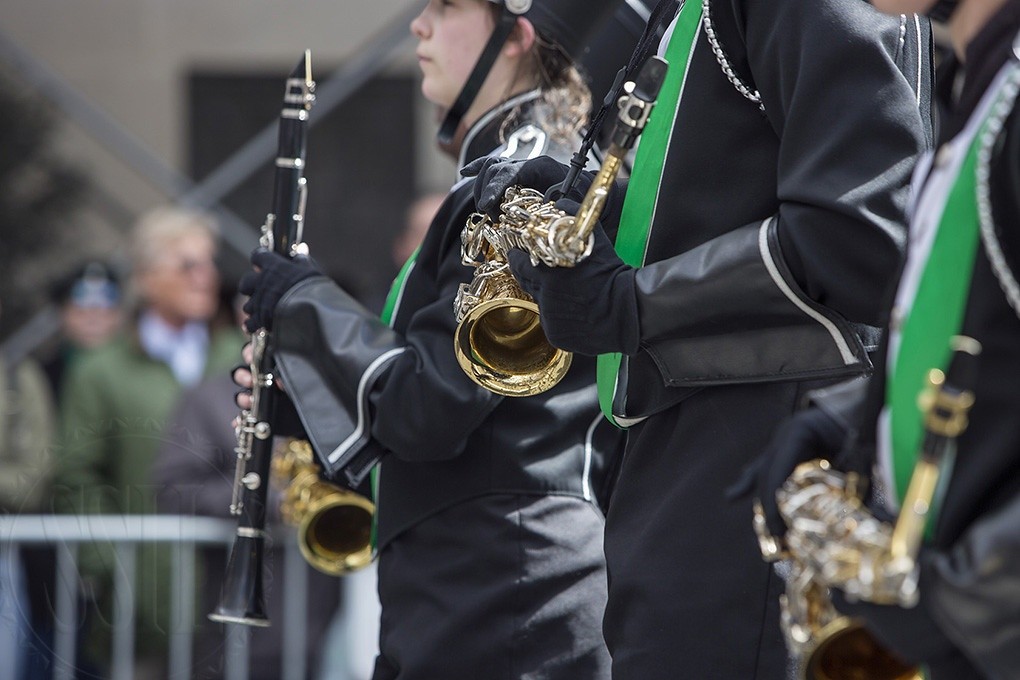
[507,197,643,358]
[831,553,988,680]
[474,154,570,228]
[751,408,849,536]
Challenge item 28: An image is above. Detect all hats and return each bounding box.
[491,0,664,114]
[50,261,121,309]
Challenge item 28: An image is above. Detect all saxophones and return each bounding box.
[754,337,983,680]
[451,54,669,396]
[270,411,377,577]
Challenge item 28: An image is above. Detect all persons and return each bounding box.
[0,194,447,680]
[235,1,1020,680]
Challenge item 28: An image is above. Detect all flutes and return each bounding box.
[204,47,315,632]
